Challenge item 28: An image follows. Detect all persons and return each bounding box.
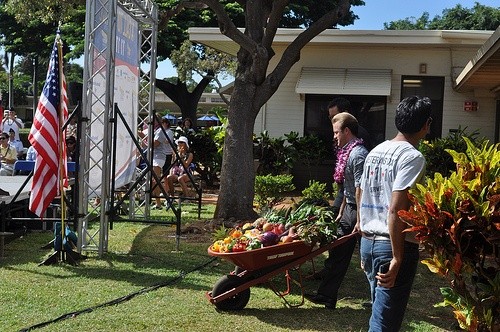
[301,98,373,311]
[352,96,434,332]
[0,109,196,211]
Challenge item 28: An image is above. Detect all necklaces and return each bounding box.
[333,139,364,183]
[0,145,10,157]
[8,117,16,126]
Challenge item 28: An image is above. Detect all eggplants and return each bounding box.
[258,231,280,246]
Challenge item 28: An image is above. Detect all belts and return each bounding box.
[362,233,390,240]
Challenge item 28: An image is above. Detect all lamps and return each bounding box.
[420,63,427,74]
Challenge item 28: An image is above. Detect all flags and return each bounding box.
[28,30,69,215]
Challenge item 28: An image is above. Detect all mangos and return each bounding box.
[242,218,293,243]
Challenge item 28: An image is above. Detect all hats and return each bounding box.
[175,136,189,148]
[9,128,14,132]
[10,107,15,111]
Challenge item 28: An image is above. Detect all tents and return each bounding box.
[163,113,221,126]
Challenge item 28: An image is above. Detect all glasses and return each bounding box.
[328,116,332,120]
[0,138,5,140]
[427,116,432,126]
[178,142,185,144]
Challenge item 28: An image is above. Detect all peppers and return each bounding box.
[211,230,260,253]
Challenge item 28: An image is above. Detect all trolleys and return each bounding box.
[205,212,362,314]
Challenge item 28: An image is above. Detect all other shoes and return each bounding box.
[0,189,9,196]
[151,206,163,210]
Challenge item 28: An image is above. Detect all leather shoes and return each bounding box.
[304,294,336,310]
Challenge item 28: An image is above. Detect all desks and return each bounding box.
[13,160,76,176]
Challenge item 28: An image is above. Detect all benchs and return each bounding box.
[112,174,202,220]
